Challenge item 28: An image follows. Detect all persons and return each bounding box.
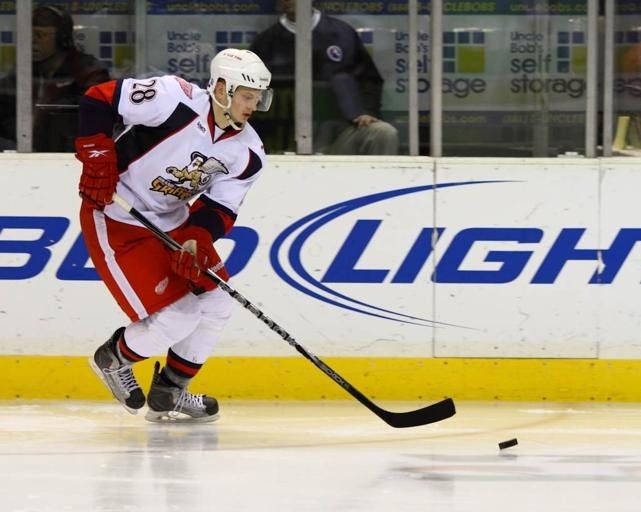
[1,7,117,152]
[248,1,400,156]
[64,48,275,424]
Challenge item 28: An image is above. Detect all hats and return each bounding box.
[206,48,271,95]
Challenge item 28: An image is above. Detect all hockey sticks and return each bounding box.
[111,192,455,428]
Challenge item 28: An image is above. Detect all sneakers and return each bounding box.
[90,327,146,419]
[147,361,219,418]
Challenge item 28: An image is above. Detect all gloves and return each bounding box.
[75,135,120,206]
[171,224,212,283]
